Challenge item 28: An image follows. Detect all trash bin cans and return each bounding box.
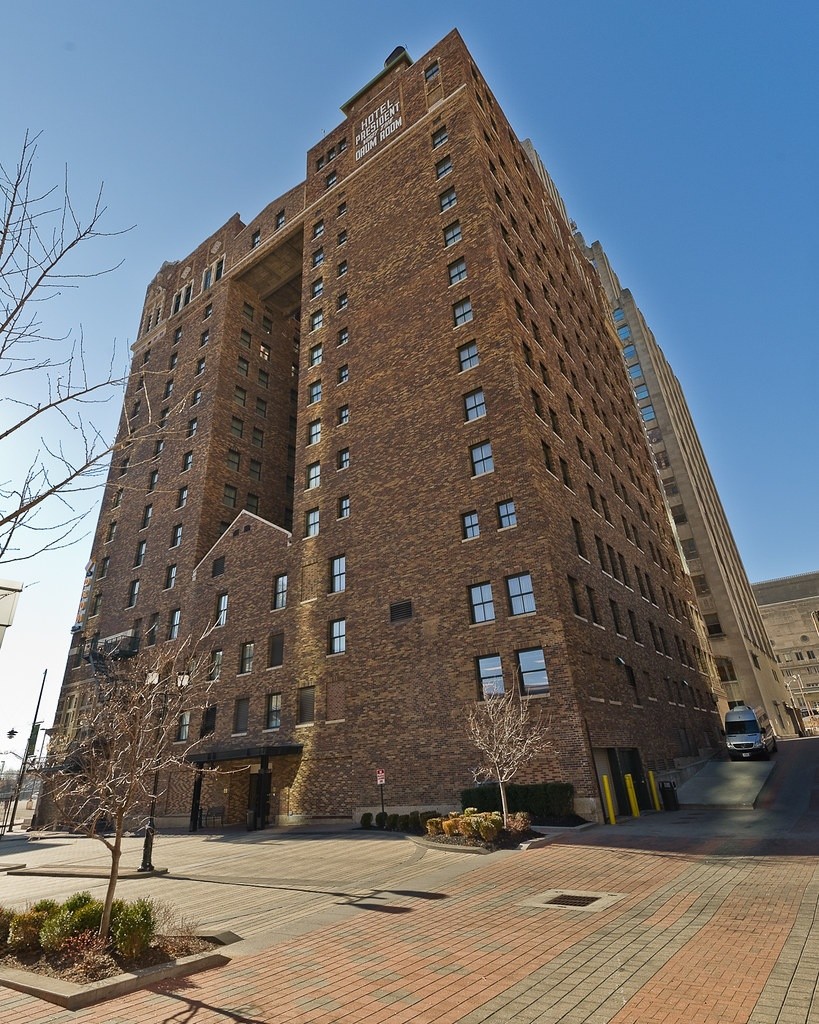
[658,779,680,811]
[246,809,257,832]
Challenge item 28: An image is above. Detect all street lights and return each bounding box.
[792,674,814,716]
[137,669,192,873]
[783,679,804,737]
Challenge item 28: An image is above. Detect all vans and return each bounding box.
[720,705,779,762]
